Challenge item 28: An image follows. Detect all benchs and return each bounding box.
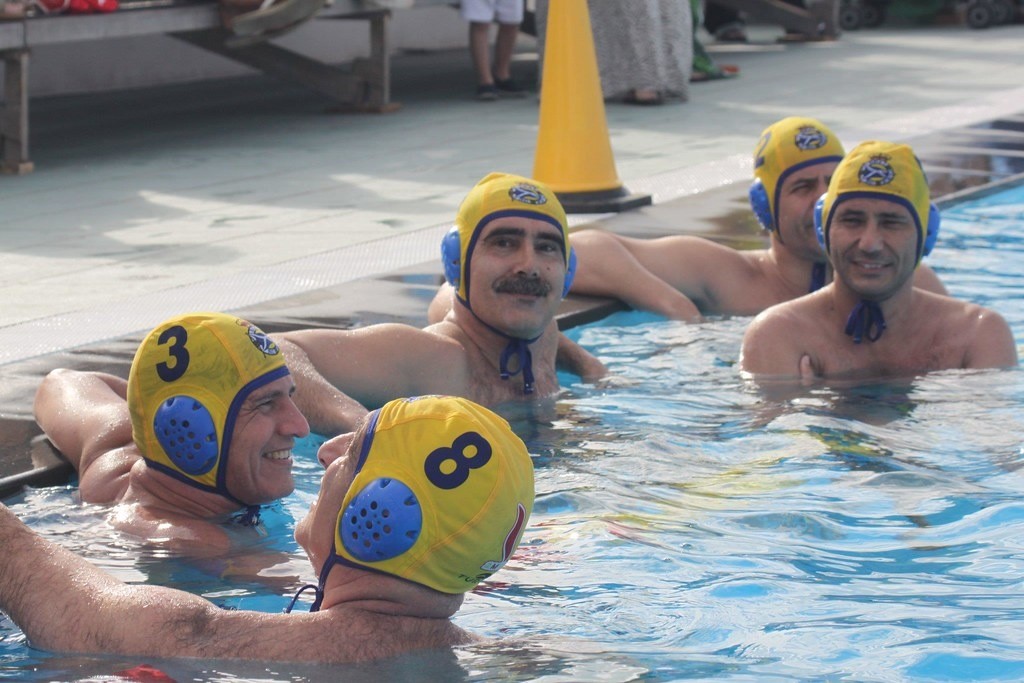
[0,0,416,174]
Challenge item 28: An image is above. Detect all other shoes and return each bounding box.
[477,84,498,100]
[623,86,665,103]
[493,78,522,95]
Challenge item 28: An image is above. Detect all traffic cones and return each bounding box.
[529,0,648,214]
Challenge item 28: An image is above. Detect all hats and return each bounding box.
[749,116,848,245]
[811,140,940,271]
[440,172,575,309]
[126,313,290,493]
[331,394,535,595]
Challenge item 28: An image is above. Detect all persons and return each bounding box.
[532,0,693,105]
[0,393,535,670]
[256,172,609,441]
[739,142,1020,393]
[460,0,527,100]
[701,0,811,44]
[30,314,321,589]
[558,115,951,324]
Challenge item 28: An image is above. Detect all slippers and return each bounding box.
[224,18,308,47]
[233,0,324,38]
